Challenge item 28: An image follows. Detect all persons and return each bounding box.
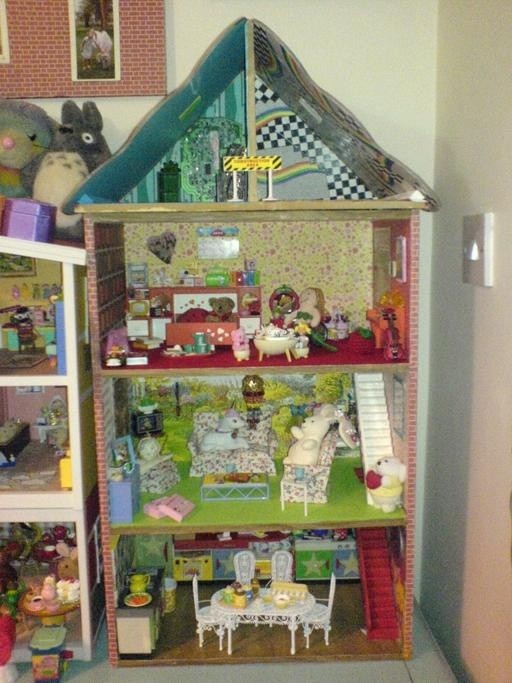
[11,283,59,301]
[79,28,113,71]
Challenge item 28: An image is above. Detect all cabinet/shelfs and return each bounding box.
[1,233,108,668]
[73,199,432,669]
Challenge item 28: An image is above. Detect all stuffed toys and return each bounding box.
[207,297,234,322]
[283,287,331,346]
[0,97,112,243]
[231,326,250,350]
[0,522,81,666]
[139,401,408,512]
